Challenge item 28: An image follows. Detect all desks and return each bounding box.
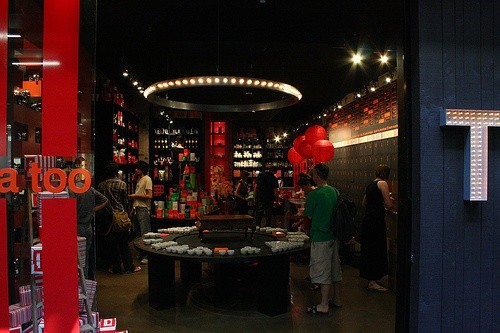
[151,208,220,228]
[197,215,255,243]
[132,228,310,317]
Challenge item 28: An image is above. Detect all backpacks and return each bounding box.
[330,188,356,235]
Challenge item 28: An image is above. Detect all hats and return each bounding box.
[240,170,249,178]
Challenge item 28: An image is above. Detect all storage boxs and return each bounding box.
[153,148,218,219]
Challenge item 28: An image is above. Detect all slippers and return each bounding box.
[306,304,331,314]
[328,300,343,308]
[368,281,387,291]
[309,283,321,290]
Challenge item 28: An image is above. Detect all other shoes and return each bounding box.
[129,267,141,272]
[140,259,148,264]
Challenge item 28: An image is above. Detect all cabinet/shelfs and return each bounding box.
[325,135,397,291]
[148,108,203,211]
[95,102,138,239]
[25,178,101,333]
[231,139,294,209]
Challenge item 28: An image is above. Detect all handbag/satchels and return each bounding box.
[112,207,131,233]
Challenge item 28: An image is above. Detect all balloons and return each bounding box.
[287,125,335,175]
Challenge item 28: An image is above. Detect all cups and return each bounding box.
[240,246,261,255]
[256,226,309,253]
[228,250,234,255]
[143,231,212,255]
[219,250,226,255]
[190,153,195,161]
[158,226,198,234]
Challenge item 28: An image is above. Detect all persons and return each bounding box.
[128,161,153,264]
[78,186,108,280]
[98,169,141,274]
[253,166,279,227]
[292,173,321,290]
[301,162,343,315]
[361,165,397,292]
[93,190,120,274]
[235,170,250,215]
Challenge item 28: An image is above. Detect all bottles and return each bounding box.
[155,128,198,148]
[226,192,237,213]
[221,195,226,214]
